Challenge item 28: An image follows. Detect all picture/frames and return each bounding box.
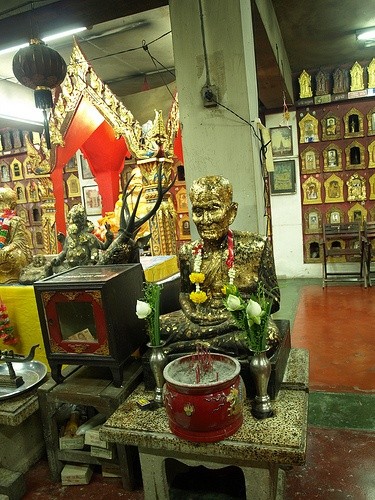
[82,185,102,216]
[80,154,94,179]
[270,160,297,196]
[269,125,294,157]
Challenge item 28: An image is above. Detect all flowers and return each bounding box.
[136,281,161,346]
[219,279,282,351]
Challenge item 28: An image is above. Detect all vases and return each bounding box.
[147,340,168,408]
[249,346,274,420]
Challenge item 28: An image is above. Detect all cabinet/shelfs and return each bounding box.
[34,263,148,388]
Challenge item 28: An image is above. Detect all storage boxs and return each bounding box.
[59,425,122,485]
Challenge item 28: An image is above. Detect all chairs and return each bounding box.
[319,220,375,290]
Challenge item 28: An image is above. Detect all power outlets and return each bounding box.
[202,85,218,108]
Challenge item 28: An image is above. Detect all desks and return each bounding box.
[37,357,143,491]
[99,347,310,500]
